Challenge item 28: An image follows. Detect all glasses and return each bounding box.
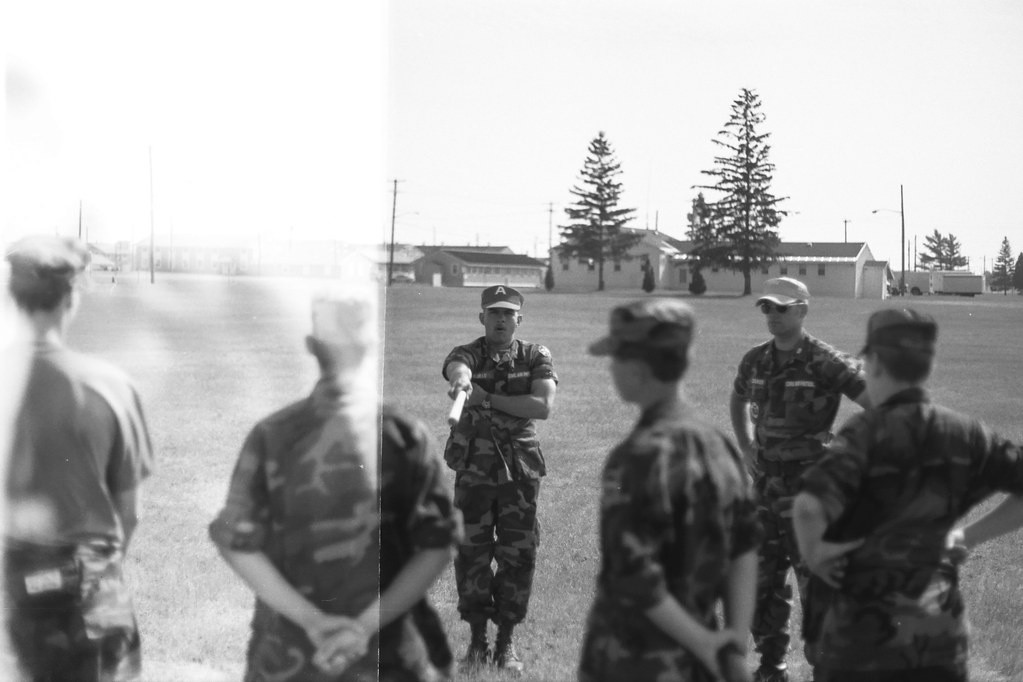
[761,304,800,314]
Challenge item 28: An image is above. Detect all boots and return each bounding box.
[466,622,491,666]
[495,623,524,675]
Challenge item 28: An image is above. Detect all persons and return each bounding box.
[574,297,762,682]
[0,233,155,682]
[728,275,871,682]
[443,284,560,677]
[208,287,466,682]
[791,309,1023,682]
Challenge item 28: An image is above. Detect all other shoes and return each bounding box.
[752,666,789,682]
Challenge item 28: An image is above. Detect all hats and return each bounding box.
[855,308,939,356]
[757,277,810,306]
[7,240,90,283]
[481,285,524,311]
[589,298,695,355]
[310,274,380,339]
